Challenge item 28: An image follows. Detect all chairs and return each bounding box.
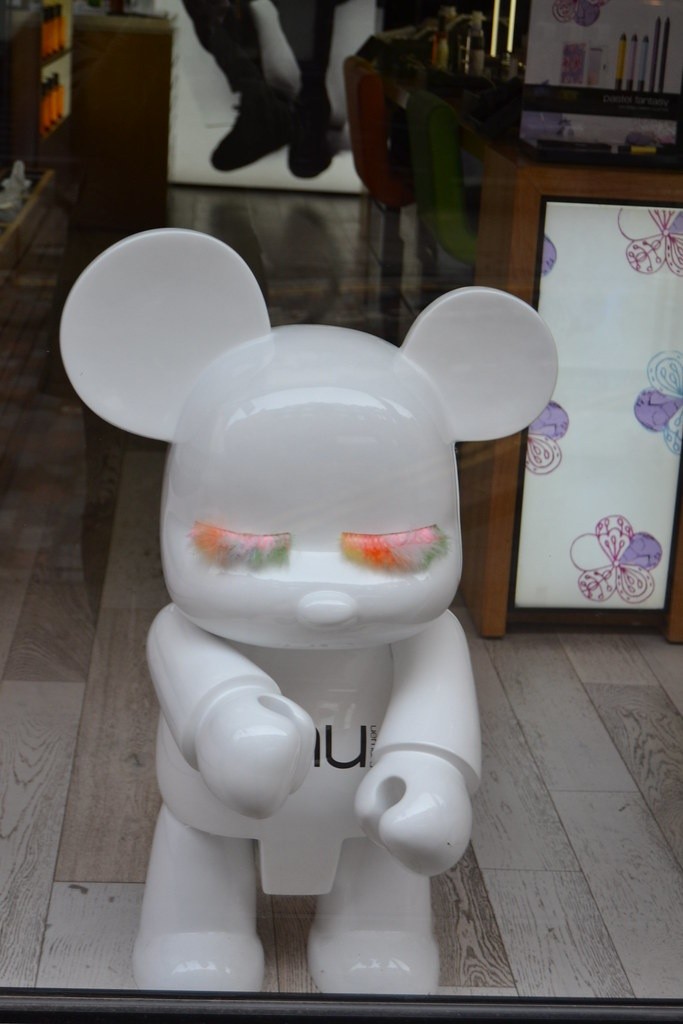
[344,55,413,206]
[405,92,476,267]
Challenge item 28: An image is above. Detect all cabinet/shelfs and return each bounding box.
[10,2,71,164]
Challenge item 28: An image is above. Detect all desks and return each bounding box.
[370,65,683,645]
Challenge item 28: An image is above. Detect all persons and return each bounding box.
[182,0,337,178]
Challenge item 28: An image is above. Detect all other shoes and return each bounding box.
[210,84,291,171]
[287,83,331,178]
